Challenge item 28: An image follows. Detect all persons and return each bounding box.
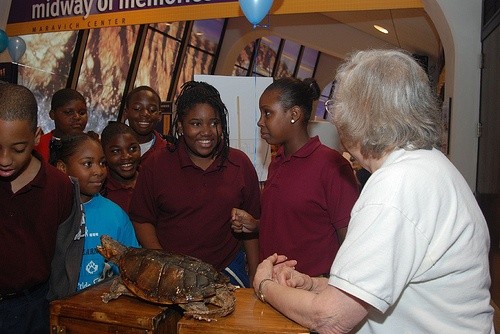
[230,46,496,334]
[0,79,260,334]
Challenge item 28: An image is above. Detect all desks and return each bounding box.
[47,268,310,334]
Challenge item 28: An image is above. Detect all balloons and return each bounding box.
[237,0,274,31]
[0,29,26,62]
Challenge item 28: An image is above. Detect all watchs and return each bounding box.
[258,279,275,305]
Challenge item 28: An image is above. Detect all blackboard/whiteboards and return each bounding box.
[193,74,272,182]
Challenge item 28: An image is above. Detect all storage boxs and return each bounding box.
[307,120,339,151]
